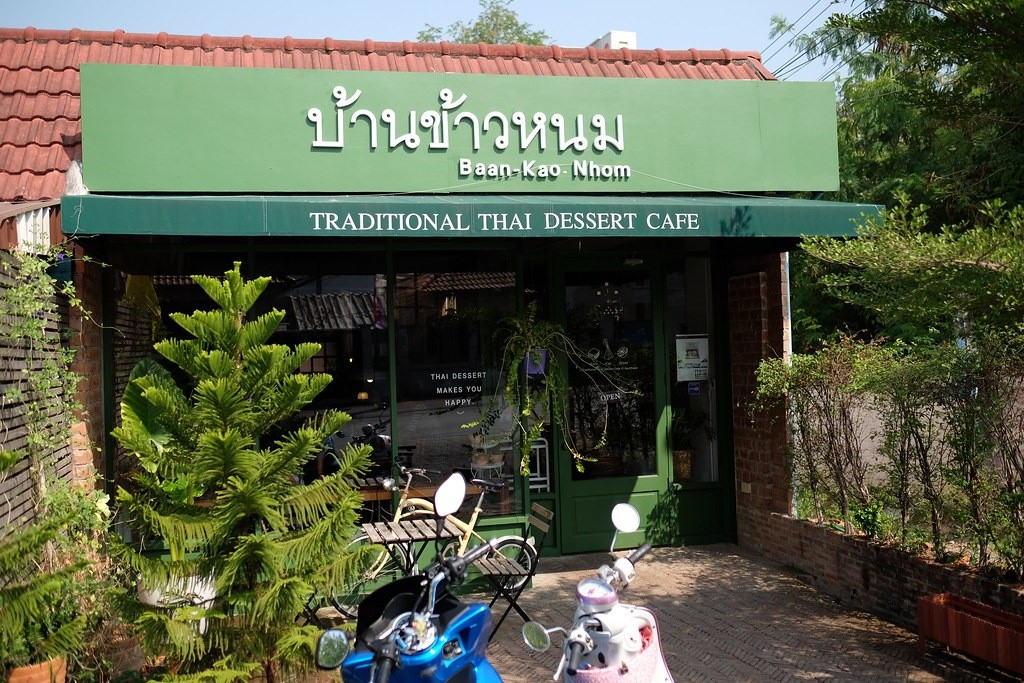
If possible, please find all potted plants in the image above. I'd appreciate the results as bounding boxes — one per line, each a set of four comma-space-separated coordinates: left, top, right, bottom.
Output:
0, 592, 78, 683
120, 360, 219, 636
461, 298, 649, 478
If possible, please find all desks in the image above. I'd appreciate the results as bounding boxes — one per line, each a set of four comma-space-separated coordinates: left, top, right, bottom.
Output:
362, 519, 465, 578
351, 476, 409, 489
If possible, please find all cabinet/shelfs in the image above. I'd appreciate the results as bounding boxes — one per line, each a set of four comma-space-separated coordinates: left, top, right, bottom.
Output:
471, 431, 552, 493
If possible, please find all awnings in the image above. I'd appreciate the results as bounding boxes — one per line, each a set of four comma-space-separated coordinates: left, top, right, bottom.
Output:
60, 193, 886, 275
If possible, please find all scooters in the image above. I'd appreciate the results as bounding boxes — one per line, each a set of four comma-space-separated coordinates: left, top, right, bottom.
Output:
521, 501, 676, 682
312, 470, 505, 683
335, 400, 391, 468
304, 430, 347, 486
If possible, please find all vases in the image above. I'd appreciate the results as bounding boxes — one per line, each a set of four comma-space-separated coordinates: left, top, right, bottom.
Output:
918, 591, 1024, 674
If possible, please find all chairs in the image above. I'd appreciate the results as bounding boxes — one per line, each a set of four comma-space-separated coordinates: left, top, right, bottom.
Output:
474, 500, 554, 643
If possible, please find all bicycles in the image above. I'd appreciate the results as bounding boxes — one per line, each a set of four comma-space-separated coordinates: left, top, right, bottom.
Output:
326, 464, 539, 619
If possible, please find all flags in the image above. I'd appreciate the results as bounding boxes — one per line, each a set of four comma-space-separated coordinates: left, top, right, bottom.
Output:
370, 297, 388, 330
